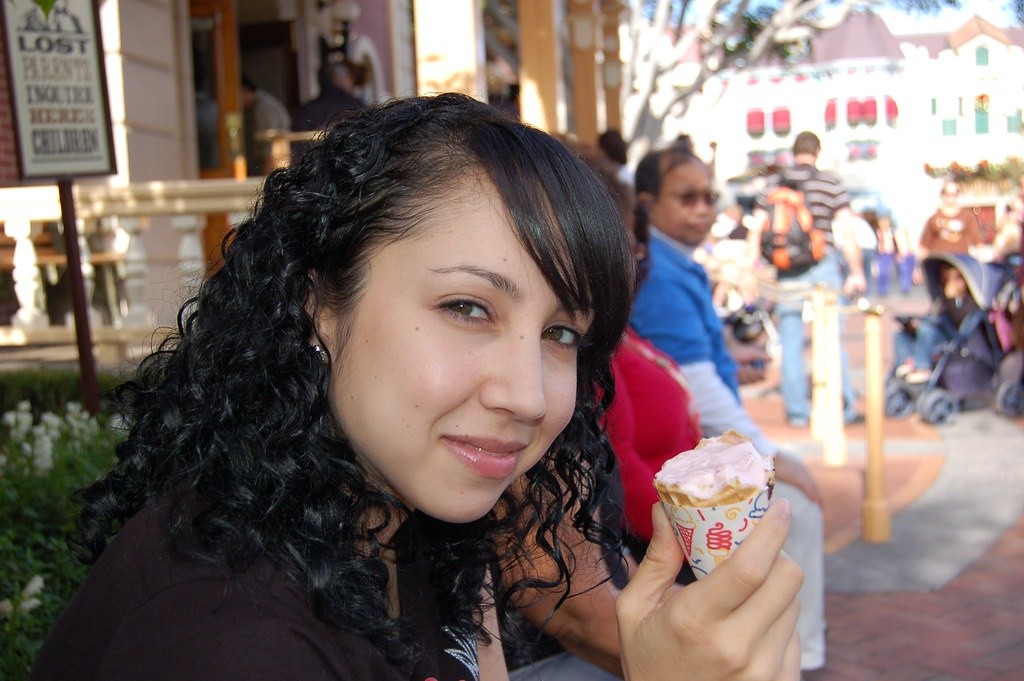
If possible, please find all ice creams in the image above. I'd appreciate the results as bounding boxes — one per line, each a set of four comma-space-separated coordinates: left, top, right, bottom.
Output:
653, 430, 775, 508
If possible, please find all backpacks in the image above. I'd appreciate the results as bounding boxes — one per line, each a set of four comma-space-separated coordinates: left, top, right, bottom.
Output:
760, 168, 826, 274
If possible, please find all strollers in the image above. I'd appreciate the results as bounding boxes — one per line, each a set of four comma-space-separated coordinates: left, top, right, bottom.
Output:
880, 251, 1024, 426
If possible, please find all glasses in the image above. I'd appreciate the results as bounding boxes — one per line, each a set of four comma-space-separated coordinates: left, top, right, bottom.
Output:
654, 189, 720, 207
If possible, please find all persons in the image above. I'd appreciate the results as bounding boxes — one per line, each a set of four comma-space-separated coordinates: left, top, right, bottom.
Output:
895, 180, 1024, 383
33, 93, 803, 681
195, 58, 371, 176
487, 128, 899, 681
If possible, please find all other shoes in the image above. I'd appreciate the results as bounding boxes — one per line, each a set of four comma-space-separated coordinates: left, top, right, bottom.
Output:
896, 362, 933, 382
848, 414, 867, 424
